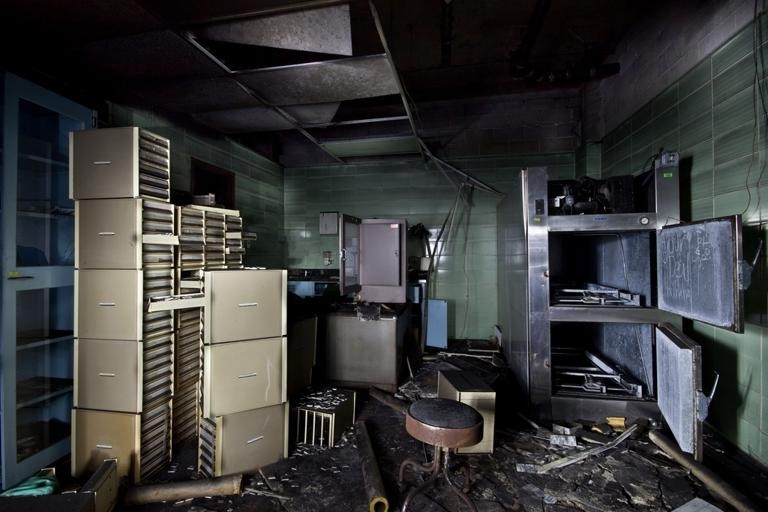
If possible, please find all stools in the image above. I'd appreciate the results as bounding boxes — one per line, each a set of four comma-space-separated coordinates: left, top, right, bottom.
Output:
396, 397, 485, 511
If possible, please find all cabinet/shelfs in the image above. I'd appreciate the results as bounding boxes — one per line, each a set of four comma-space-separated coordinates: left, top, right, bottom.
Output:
171, 204, 242, 461
68, 125, 170, 202
197, 403, 289, 480
147, 268, 287, 345
74, 269, 175, 341
74, 200, 174, 271
198, 335, 287, 420
70, 410, 170, 484
74, 335, 175, 413
